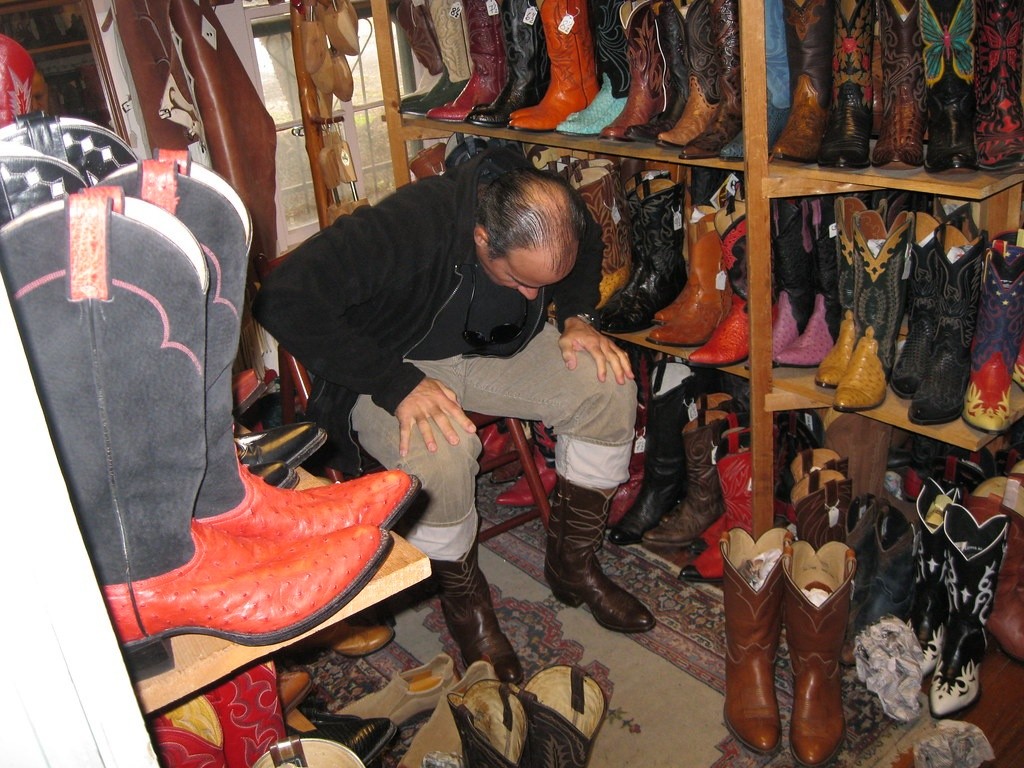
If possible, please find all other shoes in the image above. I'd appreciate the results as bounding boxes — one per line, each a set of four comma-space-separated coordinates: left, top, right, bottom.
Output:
336, 653, 456, 726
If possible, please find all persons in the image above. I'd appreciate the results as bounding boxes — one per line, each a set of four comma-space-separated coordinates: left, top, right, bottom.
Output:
250, 145, 655, 685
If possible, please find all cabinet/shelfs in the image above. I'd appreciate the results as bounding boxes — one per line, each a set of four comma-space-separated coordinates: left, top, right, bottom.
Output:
0, 0, 1024, 768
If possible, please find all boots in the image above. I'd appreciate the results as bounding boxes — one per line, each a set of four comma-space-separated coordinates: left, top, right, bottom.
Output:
430, 519, 522, 684
545, 472, 655, 632
446, 679, 528, 768
606, 350, 1024, 764
518, 664, 604, 768
396, 0, 1024, 171
479, 418, 556, 505
0, 112, 421, 681
408, 130, 1023, 434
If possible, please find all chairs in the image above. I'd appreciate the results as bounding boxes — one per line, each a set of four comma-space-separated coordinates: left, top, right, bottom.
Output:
250, 237, 554, 599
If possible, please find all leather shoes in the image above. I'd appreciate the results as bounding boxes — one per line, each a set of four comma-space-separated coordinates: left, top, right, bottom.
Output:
278, 672, 397, 763
287, 621, 395, 664
